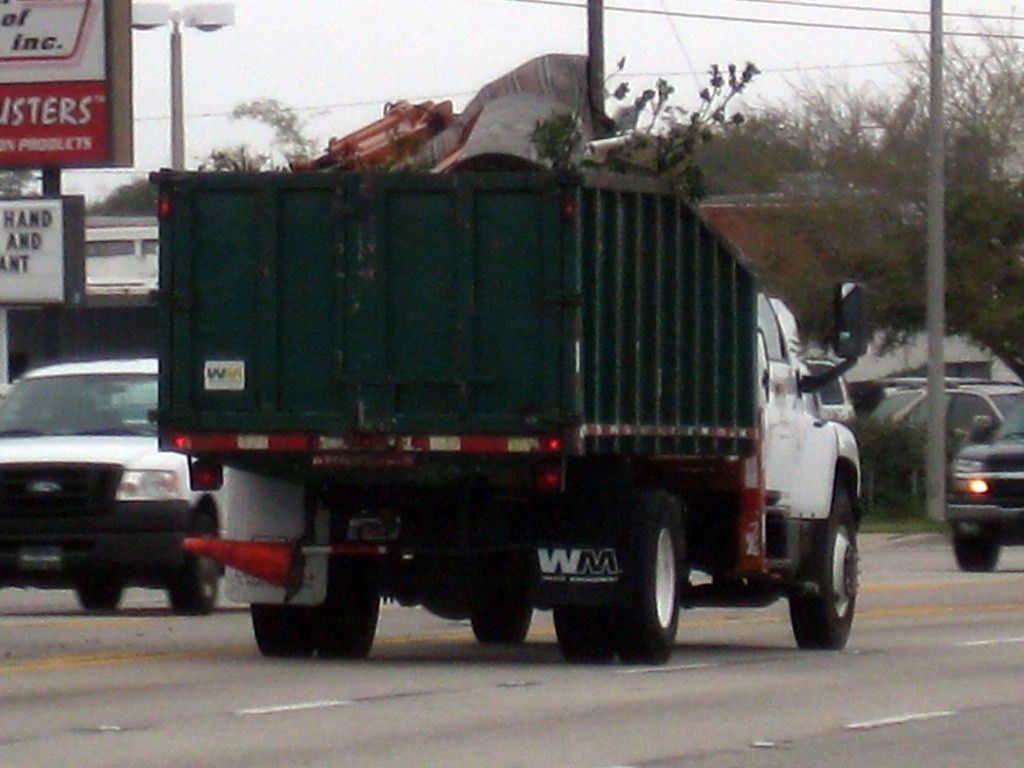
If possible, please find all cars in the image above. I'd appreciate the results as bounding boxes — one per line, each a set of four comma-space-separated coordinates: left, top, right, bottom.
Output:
0, 357, 232, 619
858, 376, 1024, 572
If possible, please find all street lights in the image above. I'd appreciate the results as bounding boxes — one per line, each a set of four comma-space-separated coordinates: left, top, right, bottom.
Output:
131, 0, 237, 171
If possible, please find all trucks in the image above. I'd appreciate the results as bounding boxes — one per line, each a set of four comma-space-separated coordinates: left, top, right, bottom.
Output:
145, 164, 871, 669
